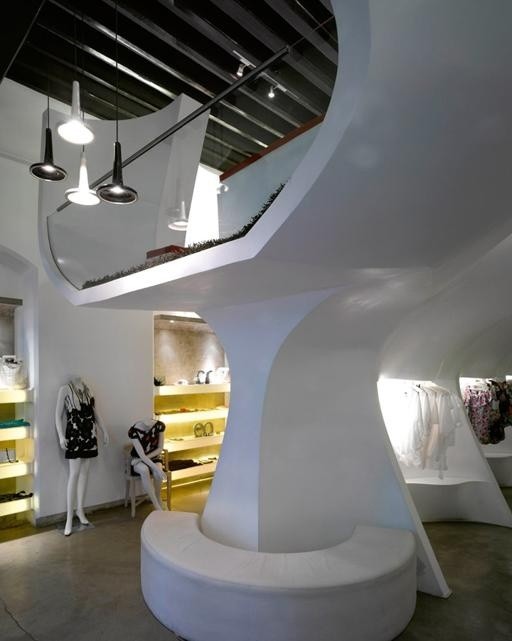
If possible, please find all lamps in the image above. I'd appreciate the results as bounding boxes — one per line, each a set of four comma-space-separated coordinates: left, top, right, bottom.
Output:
235, 50, 287, 102
28, 2, 140, 208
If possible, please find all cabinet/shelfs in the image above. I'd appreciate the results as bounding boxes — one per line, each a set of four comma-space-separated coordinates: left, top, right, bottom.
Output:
154, 378, 229, 494
1, 356, 36, 531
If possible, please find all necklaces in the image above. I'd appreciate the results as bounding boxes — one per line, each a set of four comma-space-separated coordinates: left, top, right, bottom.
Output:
70, 381, 90, 407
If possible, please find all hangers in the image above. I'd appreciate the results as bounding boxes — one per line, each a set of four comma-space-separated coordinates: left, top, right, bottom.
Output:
390, 379, 451, 400
463, 378, 511, 398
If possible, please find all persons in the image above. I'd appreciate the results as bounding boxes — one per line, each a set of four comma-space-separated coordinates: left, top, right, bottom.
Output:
54, 374, 111, 538
127, 418, 167, 512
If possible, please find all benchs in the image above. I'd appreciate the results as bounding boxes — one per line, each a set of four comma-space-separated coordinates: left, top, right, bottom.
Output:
138, 510, 419, 641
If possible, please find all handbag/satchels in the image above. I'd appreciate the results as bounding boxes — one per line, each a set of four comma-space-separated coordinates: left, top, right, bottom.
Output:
0, 447, 18, 463
0, 353, 27, 391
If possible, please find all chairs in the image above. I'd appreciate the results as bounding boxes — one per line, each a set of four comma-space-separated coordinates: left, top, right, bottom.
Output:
122, 443, 172, 518
